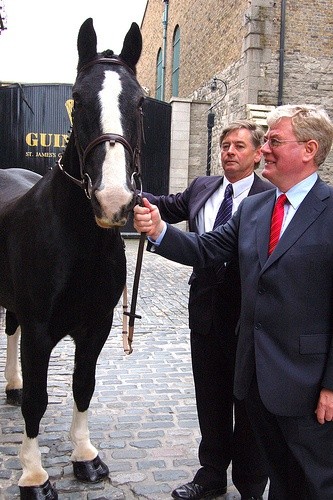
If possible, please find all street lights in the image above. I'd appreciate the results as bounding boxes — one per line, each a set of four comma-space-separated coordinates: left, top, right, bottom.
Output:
206, 77, 228, 176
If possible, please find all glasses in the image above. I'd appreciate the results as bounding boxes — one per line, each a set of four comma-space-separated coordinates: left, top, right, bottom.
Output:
262, 137, 309, 148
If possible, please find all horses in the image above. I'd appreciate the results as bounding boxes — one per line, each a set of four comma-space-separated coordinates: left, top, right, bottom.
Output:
0, 17, 147, 500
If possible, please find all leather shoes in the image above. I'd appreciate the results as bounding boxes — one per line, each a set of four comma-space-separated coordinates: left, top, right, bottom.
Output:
171, 480, 227, 500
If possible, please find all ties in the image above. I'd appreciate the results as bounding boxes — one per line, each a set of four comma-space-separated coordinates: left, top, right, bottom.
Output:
212, 184, 233, 231
267, 194, 288, 255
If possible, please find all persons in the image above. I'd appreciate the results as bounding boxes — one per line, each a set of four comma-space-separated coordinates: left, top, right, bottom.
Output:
136, 98, 332, 499
133, 121, 276, 500
